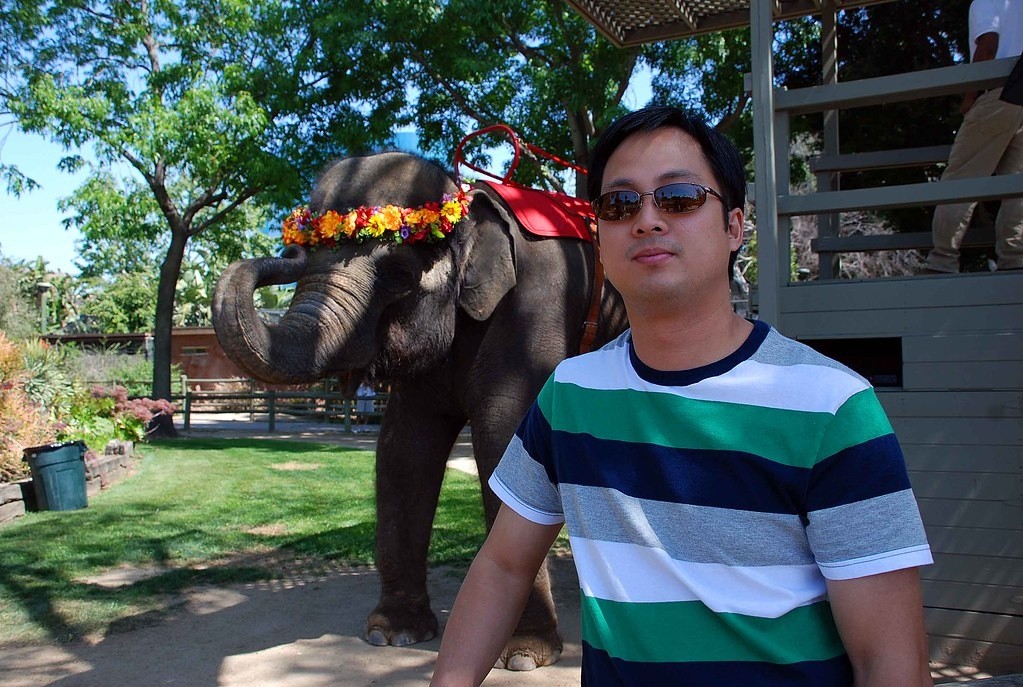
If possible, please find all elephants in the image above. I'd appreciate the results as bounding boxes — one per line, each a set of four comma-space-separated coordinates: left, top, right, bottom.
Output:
211, 152, 632, 671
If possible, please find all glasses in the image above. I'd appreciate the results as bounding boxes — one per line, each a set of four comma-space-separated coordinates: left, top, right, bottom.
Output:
591, 180, 727, 222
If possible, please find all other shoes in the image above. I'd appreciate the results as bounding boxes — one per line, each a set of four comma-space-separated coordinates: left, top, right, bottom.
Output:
924, 252, 960, 273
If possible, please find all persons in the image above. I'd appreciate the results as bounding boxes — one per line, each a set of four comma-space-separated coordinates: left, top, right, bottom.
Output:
351, 380, 376, 435
914, 2, 1020, 274
430, 103, 937, 687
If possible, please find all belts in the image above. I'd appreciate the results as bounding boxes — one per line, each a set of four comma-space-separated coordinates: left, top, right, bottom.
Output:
977, 86, 995, 98
